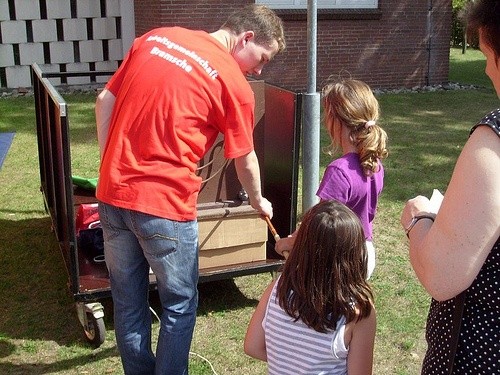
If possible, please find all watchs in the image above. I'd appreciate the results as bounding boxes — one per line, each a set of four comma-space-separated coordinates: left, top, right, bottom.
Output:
403, 212, 435, 239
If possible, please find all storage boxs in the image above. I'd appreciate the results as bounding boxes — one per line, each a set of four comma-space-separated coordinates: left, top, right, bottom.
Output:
196, 200, 269, 269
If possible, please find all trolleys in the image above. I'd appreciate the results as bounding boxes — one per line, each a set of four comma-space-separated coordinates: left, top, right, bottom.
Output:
29, 64, 304, 347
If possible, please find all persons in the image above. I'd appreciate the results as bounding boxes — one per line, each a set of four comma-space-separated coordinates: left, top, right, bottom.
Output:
243, 198, 380, 374
276, 74, 385, 281
94, 3, 286, 374
401, 0, 499, 375
